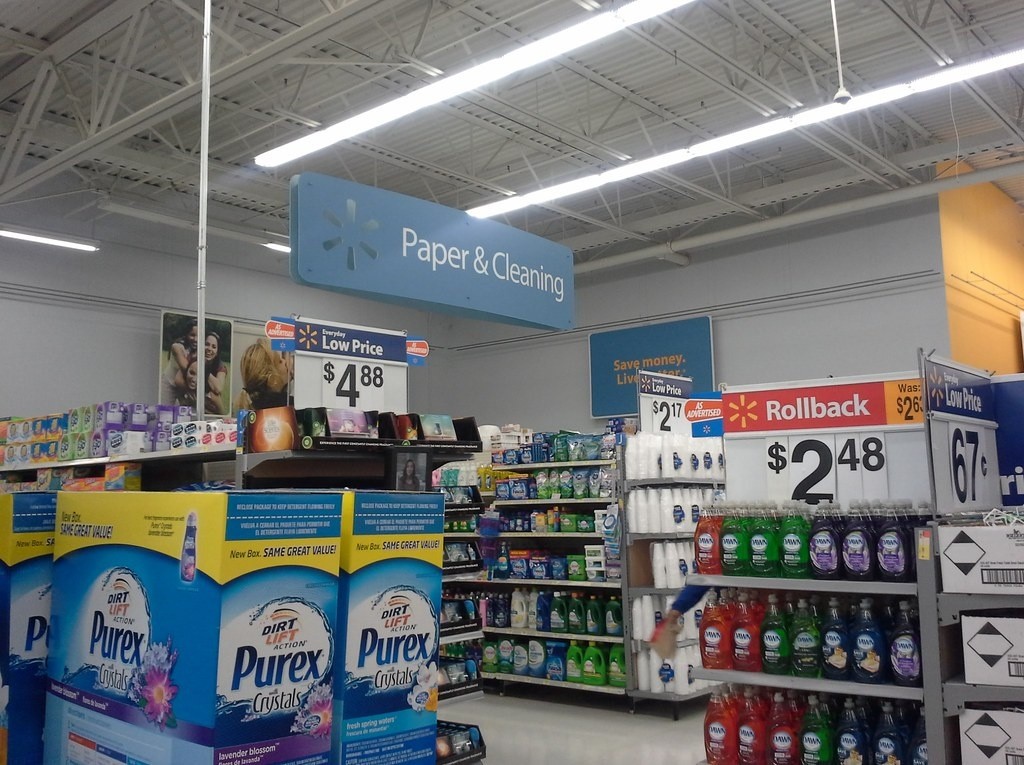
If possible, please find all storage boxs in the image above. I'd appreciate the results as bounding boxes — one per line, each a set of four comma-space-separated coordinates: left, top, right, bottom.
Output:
959, 708, 1024, 765
583, 545, 607, 582
961, 615, 1024, 687
938, 523, 1024, 595
593, 510, 608, 533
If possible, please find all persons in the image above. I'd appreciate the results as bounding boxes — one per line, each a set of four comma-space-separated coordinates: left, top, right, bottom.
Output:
343, 418, 362, 433
161, 320, 227, 414
400, 460, 420, 491
239, 340, 294, 411
666, 586, 711, 634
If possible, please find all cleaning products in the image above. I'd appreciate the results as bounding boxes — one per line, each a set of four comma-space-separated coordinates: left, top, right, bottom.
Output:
180, 512, 198, 582
624, 429, 726, 696
694, 498, 929, 765
433, 419, 627, 688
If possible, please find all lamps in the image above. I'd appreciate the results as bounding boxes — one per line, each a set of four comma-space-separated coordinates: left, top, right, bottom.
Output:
0, 222, 103, 252
466, 45, 1024, 221
253, 0, 703, 168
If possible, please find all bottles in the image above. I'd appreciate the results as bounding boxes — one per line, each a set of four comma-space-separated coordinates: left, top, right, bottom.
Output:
440, 432, 932, 765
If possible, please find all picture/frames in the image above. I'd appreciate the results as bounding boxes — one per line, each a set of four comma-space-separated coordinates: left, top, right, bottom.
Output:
158, 311, 233, 419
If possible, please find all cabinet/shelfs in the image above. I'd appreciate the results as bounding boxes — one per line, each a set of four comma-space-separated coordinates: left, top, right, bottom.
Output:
0, 315, 1024, 765
